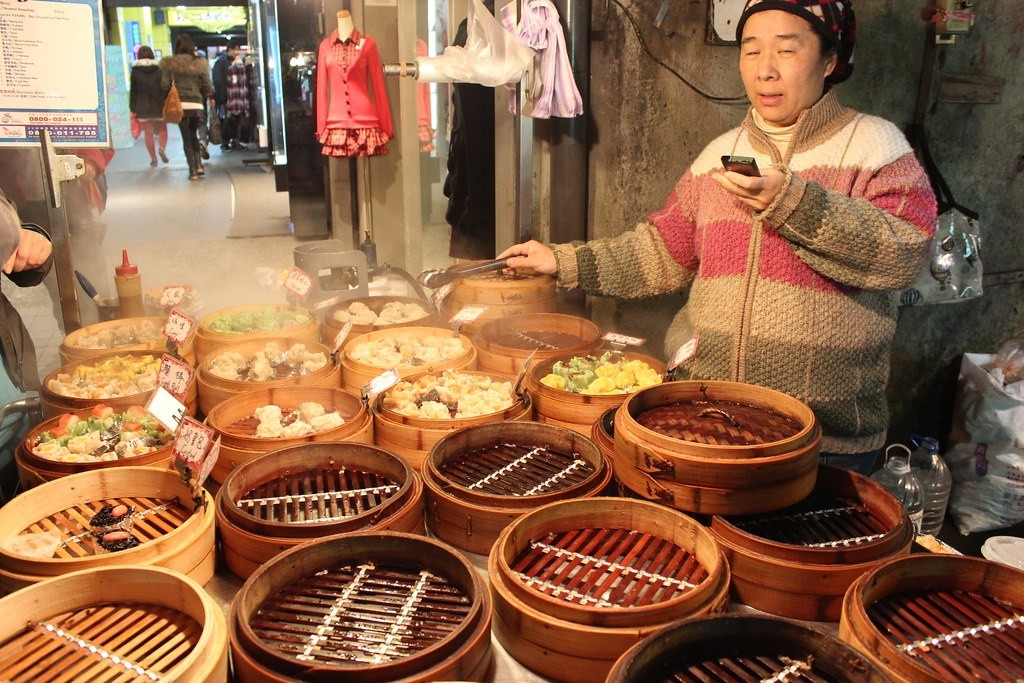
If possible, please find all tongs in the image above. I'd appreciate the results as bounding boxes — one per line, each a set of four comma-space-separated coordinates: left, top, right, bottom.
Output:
417, 254, 527, 288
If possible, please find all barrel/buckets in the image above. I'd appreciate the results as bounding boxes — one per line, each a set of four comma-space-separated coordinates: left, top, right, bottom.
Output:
97, 298, 123, 321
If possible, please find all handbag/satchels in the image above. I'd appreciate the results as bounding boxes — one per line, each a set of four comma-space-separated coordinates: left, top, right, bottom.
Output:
208, 106, 222, 145
129, 110, 140, 141
162, 75, 184, 123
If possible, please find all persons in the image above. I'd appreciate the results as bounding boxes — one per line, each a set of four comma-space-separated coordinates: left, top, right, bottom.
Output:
212, 40, 249, 152
314, 28, 395, 158
497, 0, 937, 480
161, 34, 216, 180
129, 46, 169, 167
442, 0, 497, 264
0, 179, 56, 508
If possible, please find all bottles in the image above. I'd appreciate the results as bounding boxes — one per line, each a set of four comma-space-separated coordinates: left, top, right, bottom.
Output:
870, 444, 924, 536
985, 332, 1024, 384
115, 251, 146, 317
906, 434, 952, 537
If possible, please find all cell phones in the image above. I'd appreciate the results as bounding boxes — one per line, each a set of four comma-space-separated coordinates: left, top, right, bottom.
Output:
721, 155, 761, 177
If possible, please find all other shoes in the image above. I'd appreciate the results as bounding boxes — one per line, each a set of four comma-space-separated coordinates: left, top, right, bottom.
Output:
222, 142, 249, 152
158, 150, 168, 163
197, 164, 204, 174
189, 173, 198, 180
198, 142, 210, 160
151, 160, 159, 166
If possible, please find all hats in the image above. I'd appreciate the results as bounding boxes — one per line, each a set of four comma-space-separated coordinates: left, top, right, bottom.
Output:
737, 0, 857, 85
